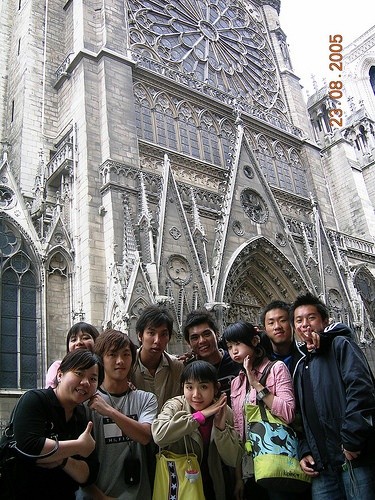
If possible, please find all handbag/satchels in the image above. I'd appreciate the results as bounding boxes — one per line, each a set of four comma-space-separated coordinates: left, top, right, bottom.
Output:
151, 431, 205, 500
244, 361, 311, 489
0, 389, 60, 476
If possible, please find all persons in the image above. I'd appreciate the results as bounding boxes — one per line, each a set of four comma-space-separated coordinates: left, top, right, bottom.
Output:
149, 359, 244, 500
127, 306, 187, 414
288, 293, 375, 500
261, 299, 322, 384
222, 320, 311, 500
87, 328, 159, 500
173, 309, 245, 410
0, 347, 105, 500
44, 321, 99, 389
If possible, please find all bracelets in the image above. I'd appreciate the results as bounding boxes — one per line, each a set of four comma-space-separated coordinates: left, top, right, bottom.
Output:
255, 388, 270, 400
58, 457, 68, 469
